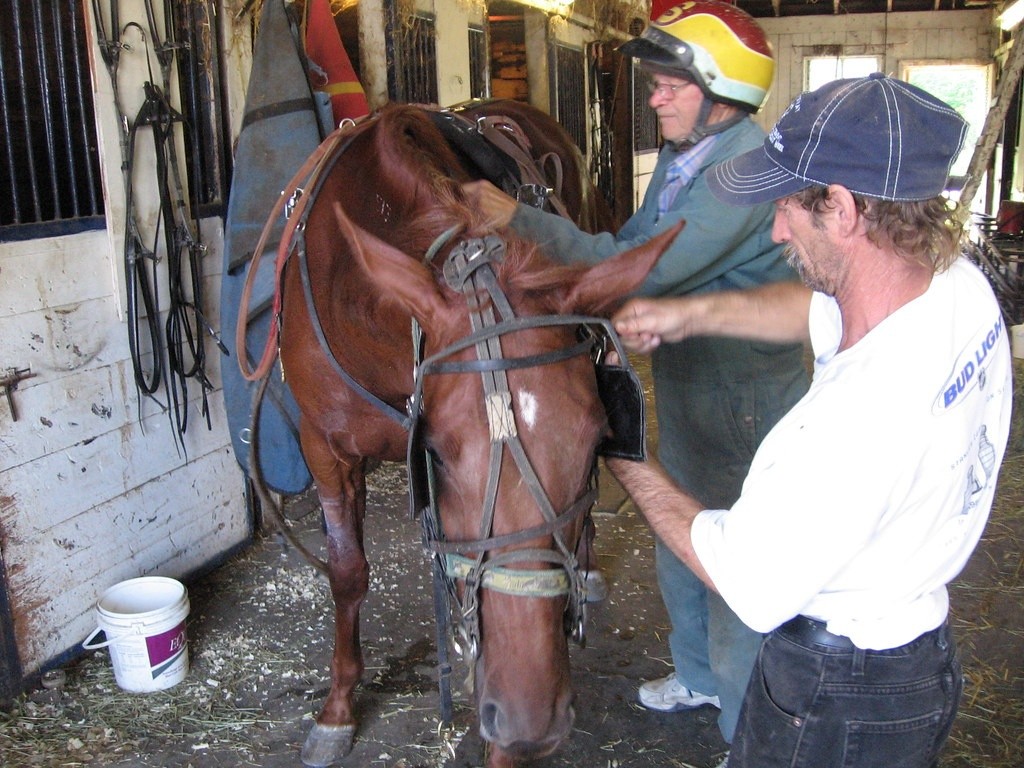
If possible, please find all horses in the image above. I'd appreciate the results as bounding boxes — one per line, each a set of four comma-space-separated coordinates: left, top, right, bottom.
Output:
277, 94, 686, 768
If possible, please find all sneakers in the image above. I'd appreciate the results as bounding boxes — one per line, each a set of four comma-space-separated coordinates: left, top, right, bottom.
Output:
638, 673, 721, 713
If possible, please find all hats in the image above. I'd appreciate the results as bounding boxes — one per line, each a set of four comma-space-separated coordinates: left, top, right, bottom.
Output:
704, 72, 970, 207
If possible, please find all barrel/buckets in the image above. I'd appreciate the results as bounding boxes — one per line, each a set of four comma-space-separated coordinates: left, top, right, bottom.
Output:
1010, 325, 1024, 359
83, 576, 193, 694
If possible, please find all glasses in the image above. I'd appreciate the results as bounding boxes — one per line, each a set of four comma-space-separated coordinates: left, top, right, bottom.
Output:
646, 80, 691, 100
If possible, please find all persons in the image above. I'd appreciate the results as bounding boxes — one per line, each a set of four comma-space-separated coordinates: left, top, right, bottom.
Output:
607, 74, 1013, 768
462, 0, 811, 768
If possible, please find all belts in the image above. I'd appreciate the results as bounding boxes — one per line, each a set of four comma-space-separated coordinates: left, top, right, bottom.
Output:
778, 613, 854, 651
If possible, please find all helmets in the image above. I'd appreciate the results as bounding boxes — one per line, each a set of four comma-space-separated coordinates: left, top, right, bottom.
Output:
613, 0, 774, 115
